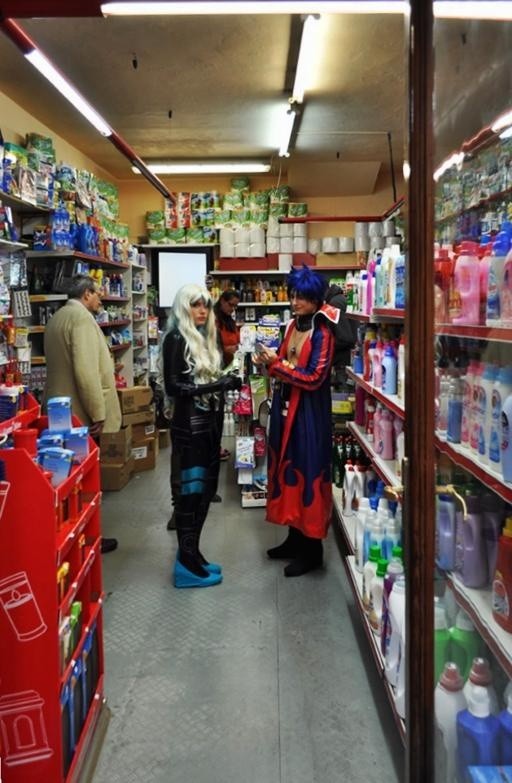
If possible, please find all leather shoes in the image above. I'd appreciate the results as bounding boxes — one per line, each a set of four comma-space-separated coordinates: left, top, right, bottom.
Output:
102, 538, 117, 553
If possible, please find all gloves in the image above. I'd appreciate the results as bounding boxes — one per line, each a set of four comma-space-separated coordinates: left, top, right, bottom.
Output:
224, 375, 242, 393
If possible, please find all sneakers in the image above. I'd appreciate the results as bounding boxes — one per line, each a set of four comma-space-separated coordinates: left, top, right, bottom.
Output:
211, 494, 221, 502
268, 544, 323, 578
220, 447, 230, 459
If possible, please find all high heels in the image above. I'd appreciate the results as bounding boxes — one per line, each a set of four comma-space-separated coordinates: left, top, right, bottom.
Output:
174, 548, 222, 588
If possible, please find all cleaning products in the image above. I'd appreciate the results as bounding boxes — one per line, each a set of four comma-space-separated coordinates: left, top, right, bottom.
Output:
335, 220, 512, 781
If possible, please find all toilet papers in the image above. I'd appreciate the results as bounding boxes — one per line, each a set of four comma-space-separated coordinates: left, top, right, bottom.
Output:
218, 218, 400, 273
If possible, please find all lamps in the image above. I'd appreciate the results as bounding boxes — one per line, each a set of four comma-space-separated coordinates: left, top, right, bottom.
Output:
0, 16, 176, 203
279, 106, 301, 157
288, 13, 320, 105
130, 158, 273, 177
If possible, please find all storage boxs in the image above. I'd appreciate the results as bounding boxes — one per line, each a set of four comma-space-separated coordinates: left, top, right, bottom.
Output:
99, 462, 133, 492
159, 428, 172, 449
132, 436, 159, 475
115, 384, 156, 424
97, 424, 134, 464
132, 424, 160, 443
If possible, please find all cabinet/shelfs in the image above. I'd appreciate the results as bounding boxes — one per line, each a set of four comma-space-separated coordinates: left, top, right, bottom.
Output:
2, 189, 30, 389
207, 260, 362, 510
1, 391, 104, 783
316, 190, 511, 783
19, 188, 159, 405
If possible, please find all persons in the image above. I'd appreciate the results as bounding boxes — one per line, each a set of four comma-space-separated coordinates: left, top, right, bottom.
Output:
43, 273, 122, 554
154, 282, 242, 588
213, 288, 244, 462
325, 284, 360, 393
250, 259, 340, 578
